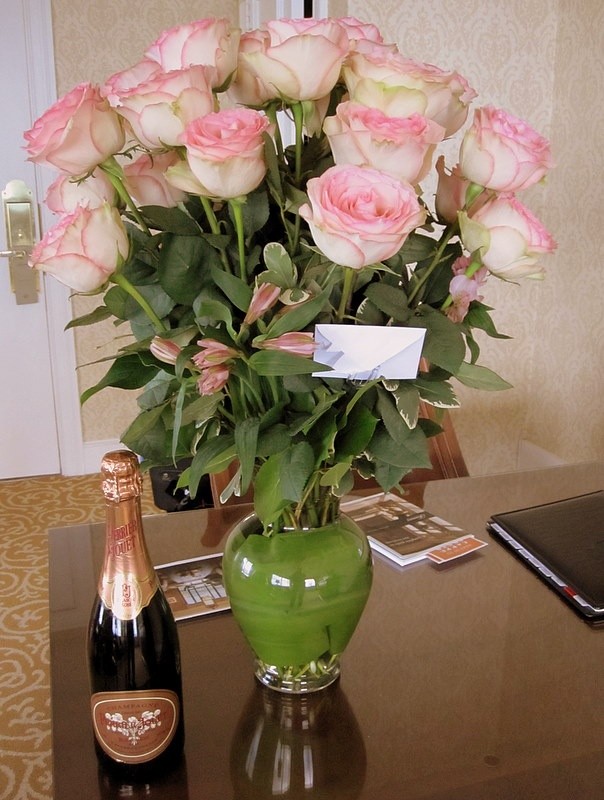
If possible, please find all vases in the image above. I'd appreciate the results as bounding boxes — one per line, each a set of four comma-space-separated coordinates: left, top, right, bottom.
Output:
222, 501, 376, 692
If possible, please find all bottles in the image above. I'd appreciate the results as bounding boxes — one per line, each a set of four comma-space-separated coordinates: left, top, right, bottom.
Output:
87, 450, 188, 785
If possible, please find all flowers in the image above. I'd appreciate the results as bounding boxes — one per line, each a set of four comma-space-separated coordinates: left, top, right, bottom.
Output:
25, 16, 557, 526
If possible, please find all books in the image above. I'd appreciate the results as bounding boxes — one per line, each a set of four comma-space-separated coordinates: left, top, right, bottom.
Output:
485, 489, 604, 630
338, 490, 473, 566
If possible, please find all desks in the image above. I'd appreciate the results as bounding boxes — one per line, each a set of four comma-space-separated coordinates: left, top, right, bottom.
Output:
47, 464, 604, 800
